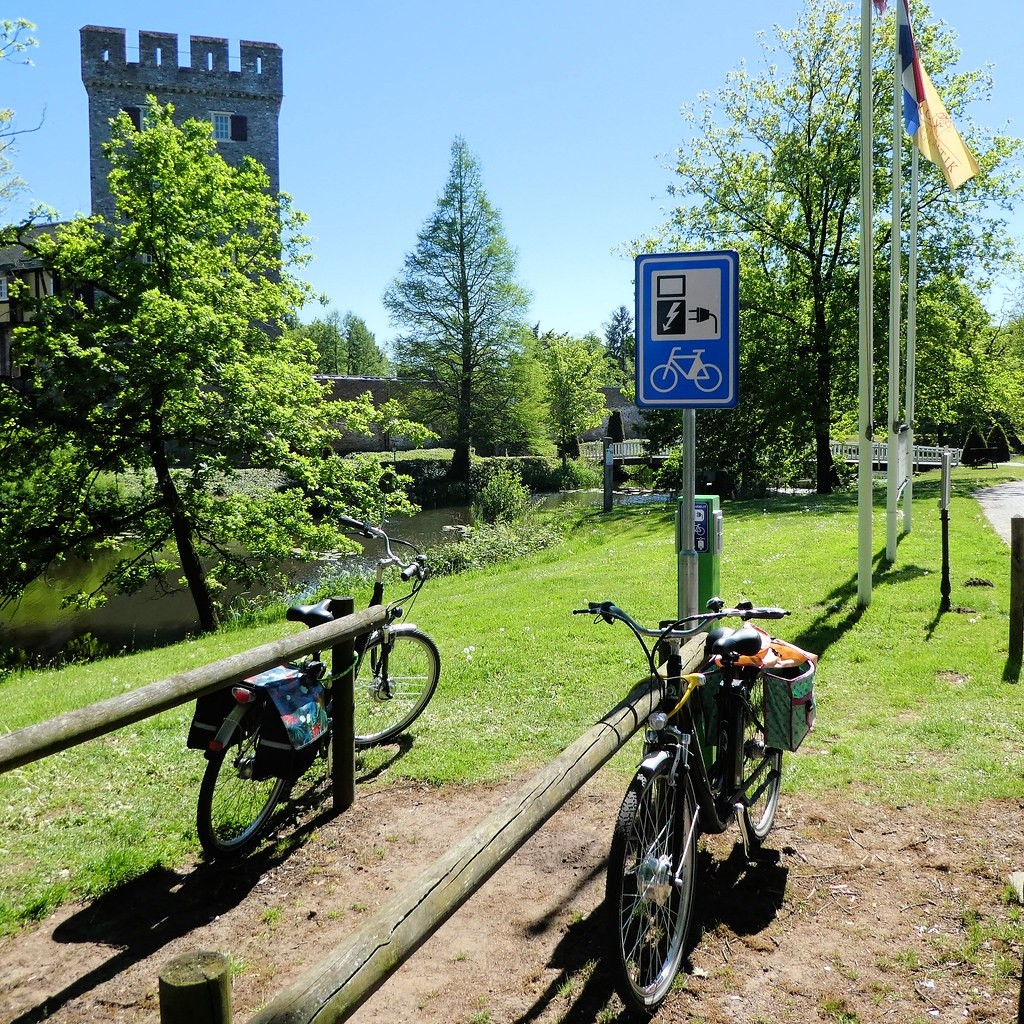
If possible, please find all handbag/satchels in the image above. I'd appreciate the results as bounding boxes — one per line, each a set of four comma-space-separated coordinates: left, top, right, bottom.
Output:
692, 639, 826, 748
188, 667, 331, 780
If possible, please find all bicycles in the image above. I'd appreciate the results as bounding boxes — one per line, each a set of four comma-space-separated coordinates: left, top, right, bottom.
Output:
570, 596, 785, 1013
193, 514, 443, 857
